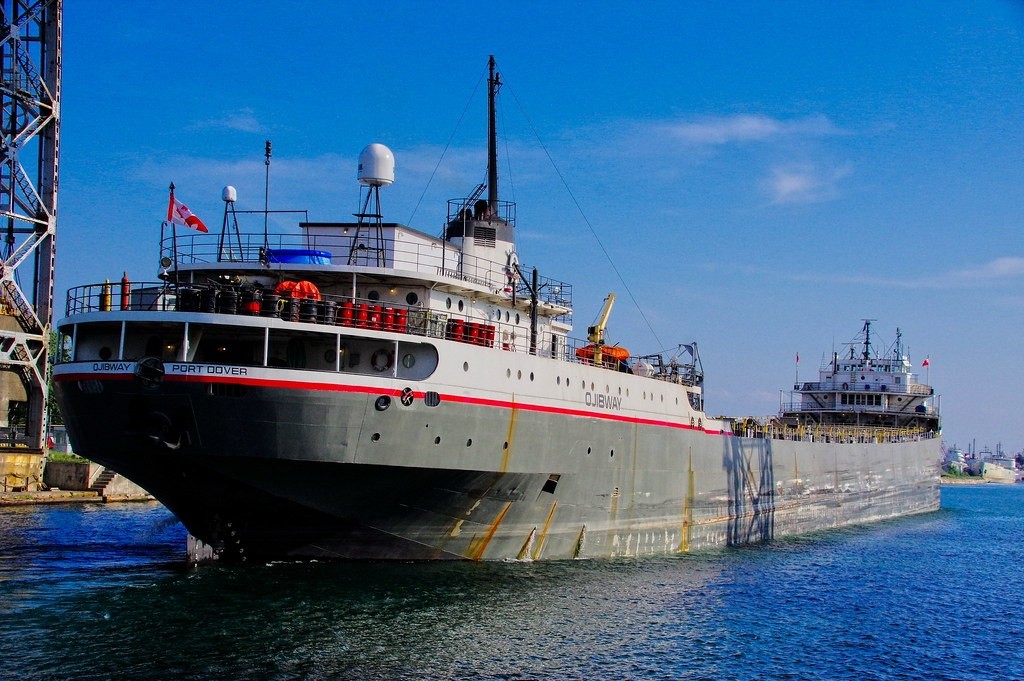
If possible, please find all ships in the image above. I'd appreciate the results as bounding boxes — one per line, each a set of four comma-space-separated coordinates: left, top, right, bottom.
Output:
49, 52, 942, 561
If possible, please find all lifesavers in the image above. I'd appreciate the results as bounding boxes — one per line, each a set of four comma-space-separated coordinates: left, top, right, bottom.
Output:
370, 348, 395, 373
509, 253, 520, 273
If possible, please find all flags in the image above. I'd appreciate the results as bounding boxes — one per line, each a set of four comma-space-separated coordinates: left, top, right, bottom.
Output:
168, 193, 210, 235
922, 359, 929, 368
796, 355, 799, 362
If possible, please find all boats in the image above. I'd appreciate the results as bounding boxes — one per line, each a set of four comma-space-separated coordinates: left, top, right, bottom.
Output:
941, 438, 1024, 484
